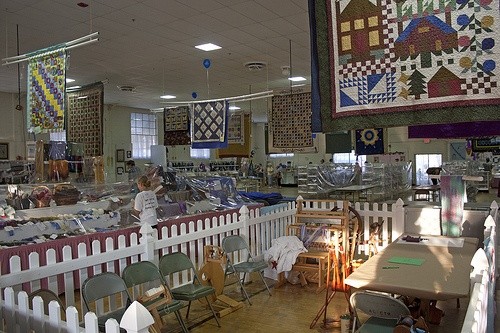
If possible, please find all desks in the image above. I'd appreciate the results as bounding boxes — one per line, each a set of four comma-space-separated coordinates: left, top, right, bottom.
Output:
274, 251, 329, 290
336, 184, 376, 202
413, 186, 440, 201
343, 233, 480, 312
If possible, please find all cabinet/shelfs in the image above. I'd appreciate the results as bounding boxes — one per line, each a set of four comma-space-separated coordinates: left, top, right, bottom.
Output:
297, 166, 318, 195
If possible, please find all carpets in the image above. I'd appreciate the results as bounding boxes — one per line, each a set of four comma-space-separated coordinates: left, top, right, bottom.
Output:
265, 90, 318, 154
26, 43, 104, 162
306, 1, 500, 153
160, 99, 252, 158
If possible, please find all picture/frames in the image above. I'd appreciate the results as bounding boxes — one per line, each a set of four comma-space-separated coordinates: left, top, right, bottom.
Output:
0, 142, 10, 160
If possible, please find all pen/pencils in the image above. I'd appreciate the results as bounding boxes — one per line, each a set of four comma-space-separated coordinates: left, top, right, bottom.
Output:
382, 266, 400, 269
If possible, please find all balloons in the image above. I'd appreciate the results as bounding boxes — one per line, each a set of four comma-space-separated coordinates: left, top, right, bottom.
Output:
203, 59, 210, 69
192, 92, 198, 99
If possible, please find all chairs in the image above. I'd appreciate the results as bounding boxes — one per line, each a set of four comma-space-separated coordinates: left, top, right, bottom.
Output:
222, 235, 273, 306
27, 289, 66, 318
122, 260, 188, 333
349, 290, 414, 333
81, 271, 155, 333
159, 252, 222, 331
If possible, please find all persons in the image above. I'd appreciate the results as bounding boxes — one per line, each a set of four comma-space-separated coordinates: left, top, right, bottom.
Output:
267, 163, 275, 188
483, 157, 493, 189
277, 163, 283, 189
131, 175, 159, 229
354, 162, 361, 183
254, 163, 264, 187
199, 161, 206, 172
209, 162, 217, 171
328, 159, 335, 166
319, 158, 327, 165
